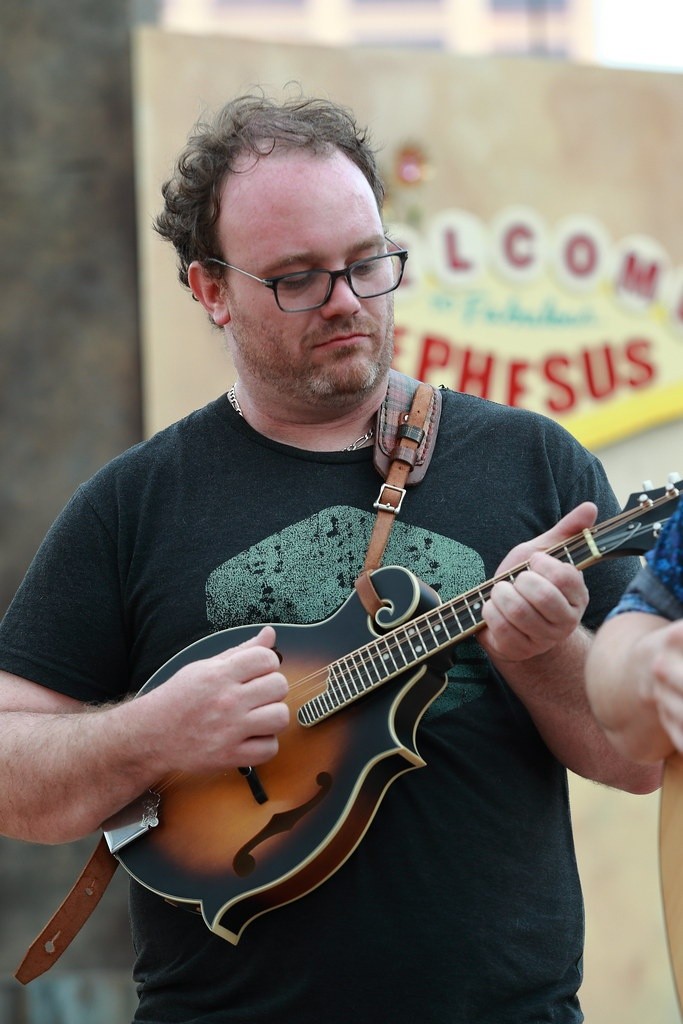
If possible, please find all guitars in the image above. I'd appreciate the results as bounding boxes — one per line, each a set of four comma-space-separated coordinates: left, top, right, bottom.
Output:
93, 474, 683, 950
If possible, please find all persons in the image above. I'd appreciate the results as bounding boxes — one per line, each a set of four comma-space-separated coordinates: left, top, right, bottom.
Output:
0, 93, 662, 1024
584, 478, 683, 1024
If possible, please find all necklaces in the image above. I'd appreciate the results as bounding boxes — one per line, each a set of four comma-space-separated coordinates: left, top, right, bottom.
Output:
230, 383, 376, 450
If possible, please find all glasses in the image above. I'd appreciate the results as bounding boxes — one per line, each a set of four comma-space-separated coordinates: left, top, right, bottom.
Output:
207, 236, 408, 312
